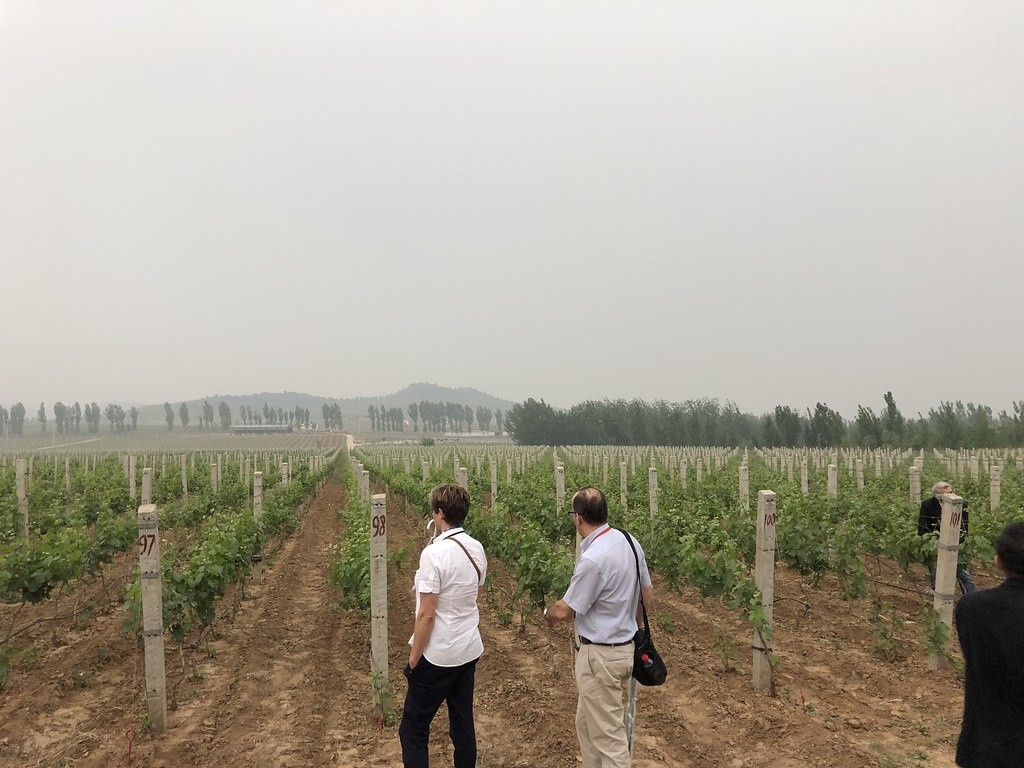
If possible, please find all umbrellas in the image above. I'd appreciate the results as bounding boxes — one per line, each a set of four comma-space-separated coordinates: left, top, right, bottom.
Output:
426, 519, 438, 546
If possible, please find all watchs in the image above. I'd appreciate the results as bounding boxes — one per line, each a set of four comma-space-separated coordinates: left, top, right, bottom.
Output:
543, 607, 550, 621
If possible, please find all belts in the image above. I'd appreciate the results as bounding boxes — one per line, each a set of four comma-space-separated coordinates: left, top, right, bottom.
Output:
578, 636, 631, 646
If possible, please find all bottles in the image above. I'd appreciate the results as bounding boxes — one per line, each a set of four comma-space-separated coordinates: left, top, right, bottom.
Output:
641, 654, 665, 685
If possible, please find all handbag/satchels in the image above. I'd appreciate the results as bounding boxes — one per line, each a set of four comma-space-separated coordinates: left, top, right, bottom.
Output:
632, 629, 668, 686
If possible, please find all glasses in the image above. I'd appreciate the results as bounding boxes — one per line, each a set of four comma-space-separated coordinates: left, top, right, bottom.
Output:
568, 511, 582, 518
428, 508, 435, 514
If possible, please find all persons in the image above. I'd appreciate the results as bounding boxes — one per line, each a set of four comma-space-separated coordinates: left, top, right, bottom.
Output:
544, 487, 653, 768
398, 482, 488, 768
954, 522, 1024, 768
918, 482, 978, 595
